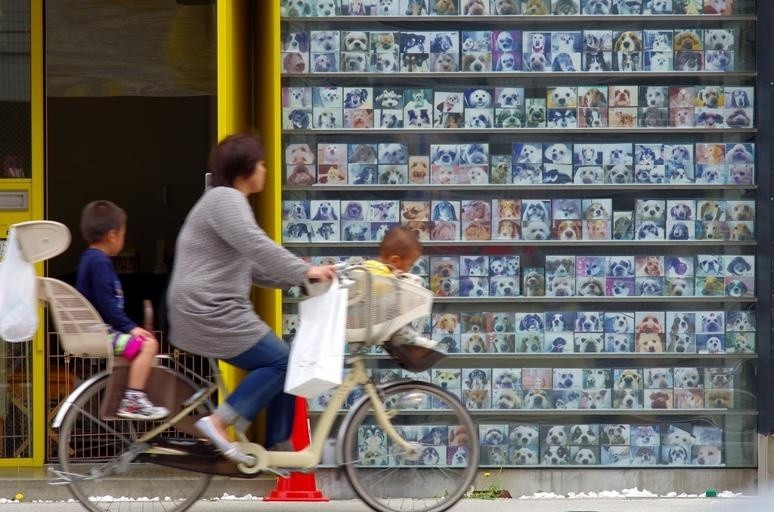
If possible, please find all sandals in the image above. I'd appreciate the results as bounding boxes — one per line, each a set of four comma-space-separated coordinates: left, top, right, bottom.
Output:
193, 416, 238, 459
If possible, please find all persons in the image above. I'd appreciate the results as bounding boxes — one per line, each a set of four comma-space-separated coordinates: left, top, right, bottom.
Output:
166, 133, 338, 462
75, 200, 170, 419
349, 226, 439, 347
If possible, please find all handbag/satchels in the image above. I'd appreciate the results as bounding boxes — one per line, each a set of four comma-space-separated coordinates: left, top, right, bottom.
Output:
0, 228, 38, 343
283, 272, 348, 399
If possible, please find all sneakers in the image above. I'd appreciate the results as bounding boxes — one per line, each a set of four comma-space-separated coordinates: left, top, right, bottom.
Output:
114, 390, 170, 419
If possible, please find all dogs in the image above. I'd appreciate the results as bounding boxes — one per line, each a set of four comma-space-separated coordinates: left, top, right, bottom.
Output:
281, 0, 756, 466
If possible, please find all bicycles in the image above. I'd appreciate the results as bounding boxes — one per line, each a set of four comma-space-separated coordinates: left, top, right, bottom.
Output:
52, 264, 481, 512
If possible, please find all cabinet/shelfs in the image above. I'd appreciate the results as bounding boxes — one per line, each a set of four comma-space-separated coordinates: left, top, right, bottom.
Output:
280, 0, 758, 469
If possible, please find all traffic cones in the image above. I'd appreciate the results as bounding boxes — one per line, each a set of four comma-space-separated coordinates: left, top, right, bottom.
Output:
262, 396, 330, 501
